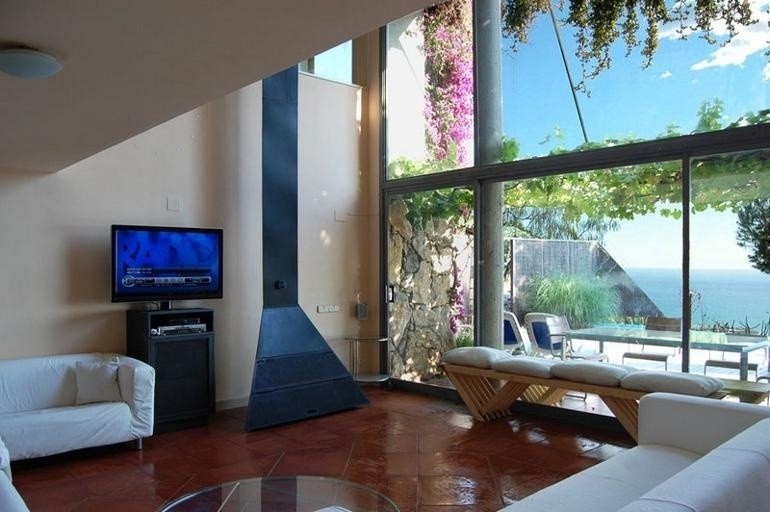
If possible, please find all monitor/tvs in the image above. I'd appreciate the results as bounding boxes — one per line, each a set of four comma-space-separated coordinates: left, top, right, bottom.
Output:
109, 223, 223, 310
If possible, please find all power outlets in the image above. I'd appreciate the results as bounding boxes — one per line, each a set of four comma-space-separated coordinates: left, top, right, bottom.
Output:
334, 208, 351, 222
167, 195, 183, 211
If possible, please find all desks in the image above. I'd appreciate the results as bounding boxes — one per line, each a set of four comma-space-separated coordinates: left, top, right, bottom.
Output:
156, 475, 400, 512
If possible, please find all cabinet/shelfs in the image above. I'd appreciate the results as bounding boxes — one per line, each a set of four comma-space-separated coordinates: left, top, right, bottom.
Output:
344, 334, 391, 386
126, 306, 217, 434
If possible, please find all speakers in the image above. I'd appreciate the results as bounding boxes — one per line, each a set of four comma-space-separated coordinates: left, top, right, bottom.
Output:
356, 303, 368, 320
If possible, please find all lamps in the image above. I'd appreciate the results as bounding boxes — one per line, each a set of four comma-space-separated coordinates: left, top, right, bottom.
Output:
1, 46, 63, 80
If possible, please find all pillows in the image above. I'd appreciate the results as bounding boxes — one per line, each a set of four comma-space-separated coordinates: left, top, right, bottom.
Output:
439, 342, 724, 447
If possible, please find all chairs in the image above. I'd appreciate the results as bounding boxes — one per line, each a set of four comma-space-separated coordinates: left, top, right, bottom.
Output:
503, 310, 770, 384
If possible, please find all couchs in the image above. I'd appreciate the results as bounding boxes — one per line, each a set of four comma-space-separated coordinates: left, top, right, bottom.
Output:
0, 350, 156, 462
494, 391, 770, 512
